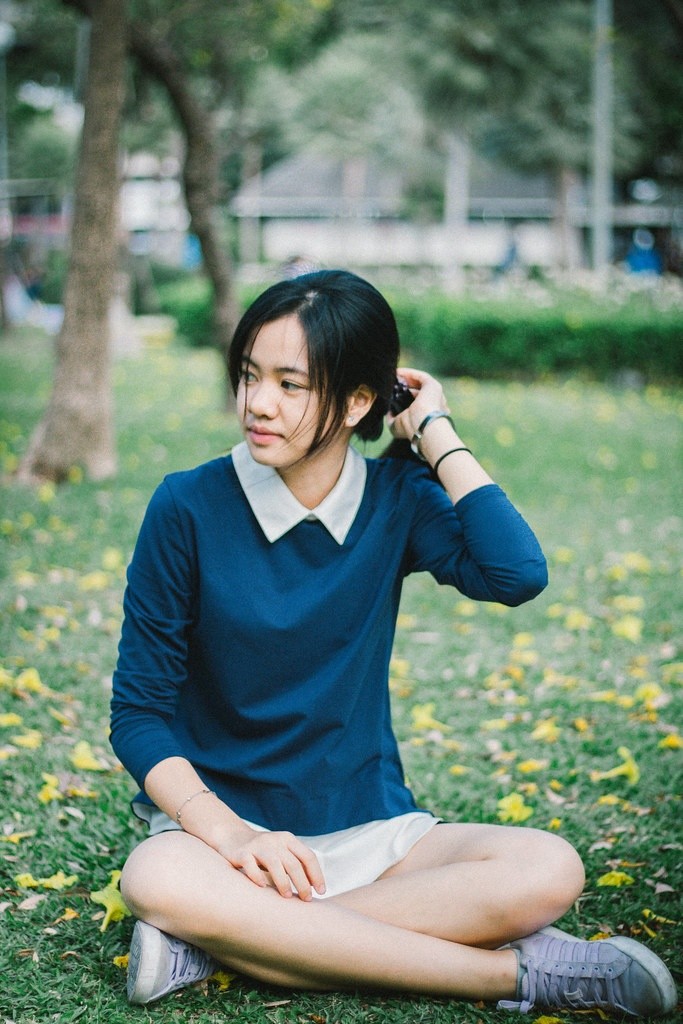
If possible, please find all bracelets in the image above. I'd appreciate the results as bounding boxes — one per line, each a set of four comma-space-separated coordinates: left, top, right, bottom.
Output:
409, 410, 456, 462
433, 446, 472, 484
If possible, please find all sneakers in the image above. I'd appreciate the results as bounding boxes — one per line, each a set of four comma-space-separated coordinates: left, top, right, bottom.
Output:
126, 918, 225, 1005
496, 924, 678, 1020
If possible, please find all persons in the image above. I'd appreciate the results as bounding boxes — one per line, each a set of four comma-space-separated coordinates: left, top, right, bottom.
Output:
110, 268, 680, 1022
